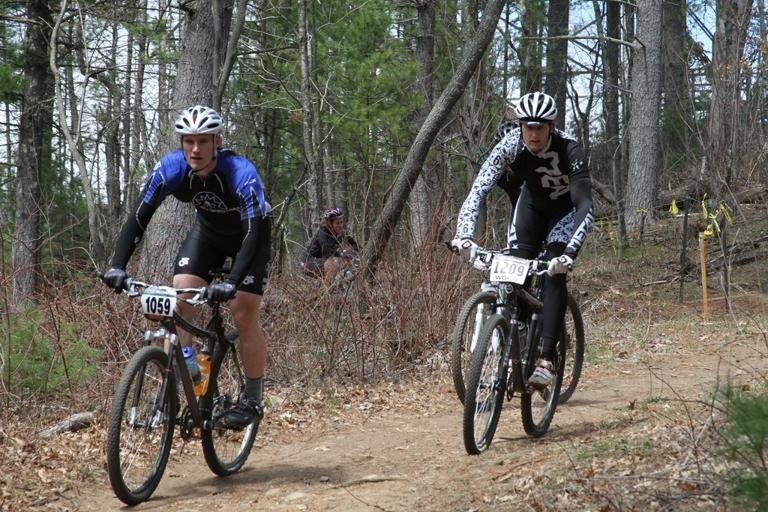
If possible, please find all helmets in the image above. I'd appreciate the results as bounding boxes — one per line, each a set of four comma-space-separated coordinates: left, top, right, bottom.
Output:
175, 104, 222, 136
515, 91, 558, 125
323, 207, 344, 220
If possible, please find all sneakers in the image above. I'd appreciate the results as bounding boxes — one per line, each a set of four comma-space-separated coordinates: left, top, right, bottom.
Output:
528, 358, 556, 384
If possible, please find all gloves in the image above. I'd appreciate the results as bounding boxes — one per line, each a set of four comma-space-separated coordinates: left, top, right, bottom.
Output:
205, 282, 236, 302
451, 238, 478, 264
546, 255, 573, 277
103, 267, 126, 294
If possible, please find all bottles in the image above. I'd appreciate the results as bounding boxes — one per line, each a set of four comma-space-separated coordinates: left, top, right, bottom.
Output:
182, 346, 202, 387
194, 347, 212, 396
518, 321, 527, 355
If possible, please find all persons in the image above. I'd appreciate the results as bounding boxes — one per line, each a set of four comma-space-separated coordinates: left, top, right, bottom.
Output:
449, 88, 598, 392
98, 103, 275, 430
297, 204, 361, 296
490, 117, 565, 361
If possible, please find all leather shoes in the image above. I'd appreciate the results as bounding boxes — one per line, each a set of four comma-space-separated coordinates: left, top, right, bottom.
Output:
227, 401, 265, 424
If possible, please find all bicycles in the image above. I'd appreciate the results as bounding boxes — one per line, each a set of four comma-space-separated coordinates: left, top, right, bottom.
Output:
95, 270, 264, 505
444, 238, 585, 454
